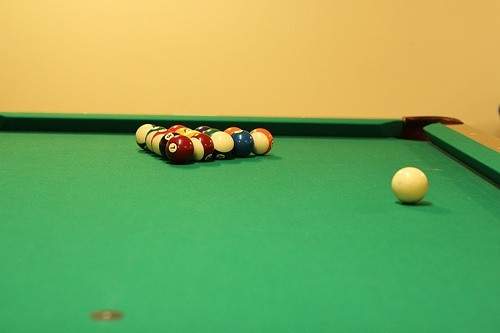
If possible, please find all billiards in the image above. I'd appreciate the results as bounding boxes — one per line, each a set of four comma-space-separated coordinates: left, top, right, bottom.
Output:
135, 123, 275, 166
391, 167, 429, 205
